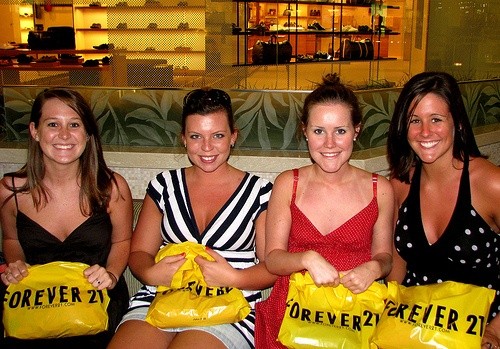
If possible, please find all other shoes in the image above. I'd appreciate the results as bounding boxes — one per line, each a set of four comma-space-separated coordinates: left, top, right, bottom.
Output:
297, 51, 327, 61
232, 22, 242, 32
358, 23, 373, 32
253, 8, 320, 30
16, 0, 191, 66
375, 24, 393, 32
306, 22, 325, 29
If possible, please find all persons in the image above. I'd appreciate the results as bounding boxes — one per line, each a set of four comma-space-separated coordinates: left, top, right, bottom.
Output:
0, 86, 133, 349
379, 70, 500, 349
106, 85, 274, 349
255, 72, 396, 349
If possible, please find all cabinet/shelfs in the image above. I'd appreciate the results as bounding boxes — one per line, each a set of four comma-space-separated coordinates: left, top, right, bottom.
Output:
0, 0, 400, 86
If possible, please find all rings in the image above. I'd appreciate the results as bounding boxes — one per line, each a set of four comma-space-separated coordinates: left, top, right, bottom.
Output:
95, 278, 101, 285
4, 272, 12, 278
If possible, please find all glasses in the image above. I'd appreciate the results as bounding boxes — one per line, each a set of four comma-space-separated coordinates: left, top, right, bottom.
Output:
183, 88, 231, 109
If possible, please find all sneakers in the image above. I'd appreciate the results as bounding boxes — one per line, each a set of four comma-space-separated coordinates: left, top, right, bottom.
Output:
341, 24, 358, 31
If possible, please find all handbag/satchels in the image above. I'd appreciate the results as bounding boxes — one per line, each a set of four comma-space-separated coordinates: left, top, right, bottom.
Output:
276, 267, 496, 348
3, 261, 110, 338
340, 35, 374, 59
144, 240, 251, 329
